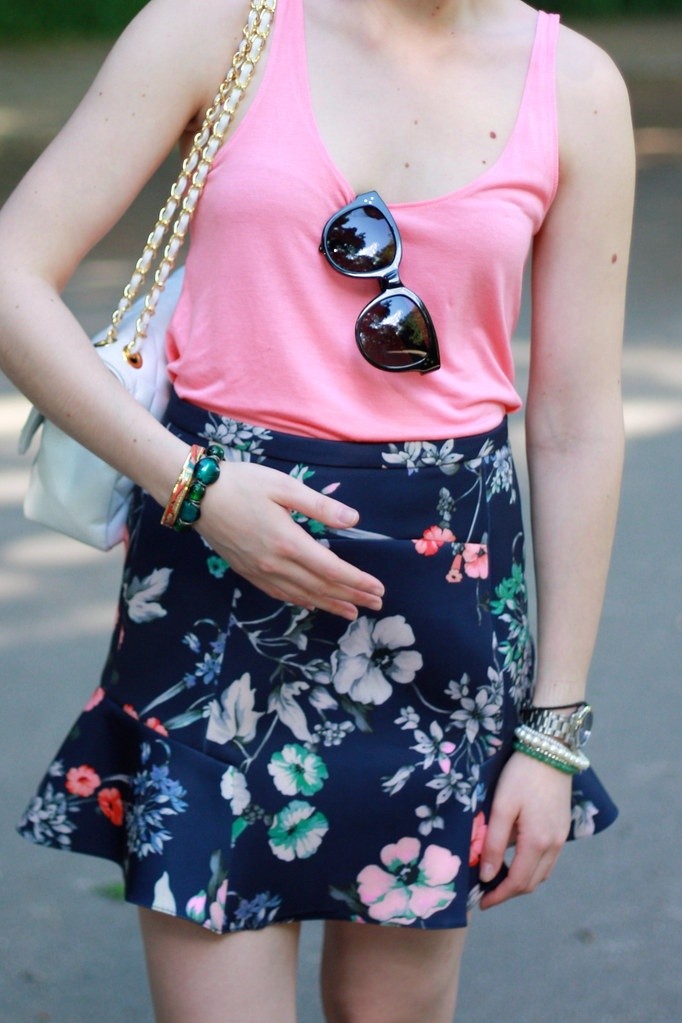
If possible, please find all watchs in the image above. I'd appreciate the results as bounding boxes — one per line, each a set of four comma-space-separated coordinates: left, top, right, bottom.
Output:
524, 705, 593, 751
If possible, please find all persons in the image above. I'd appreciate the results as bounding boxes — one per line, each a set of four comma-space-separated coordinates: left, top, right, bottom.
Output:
0, 0, 636, 1023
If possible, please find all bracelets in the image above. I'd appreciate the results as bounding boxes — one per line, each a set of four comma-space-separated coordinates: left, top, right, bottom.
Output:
512, 702, 590, 775
161, 445, 225, 532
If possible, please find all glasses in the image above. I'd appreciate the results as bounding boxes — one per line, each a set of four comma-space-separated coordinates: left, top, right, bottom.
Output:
318, 190, 440, 376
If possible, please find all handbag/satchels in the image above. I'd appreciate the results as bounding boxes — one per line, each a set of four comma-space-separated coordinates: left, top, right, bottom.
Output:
17, 265, 184, 552
